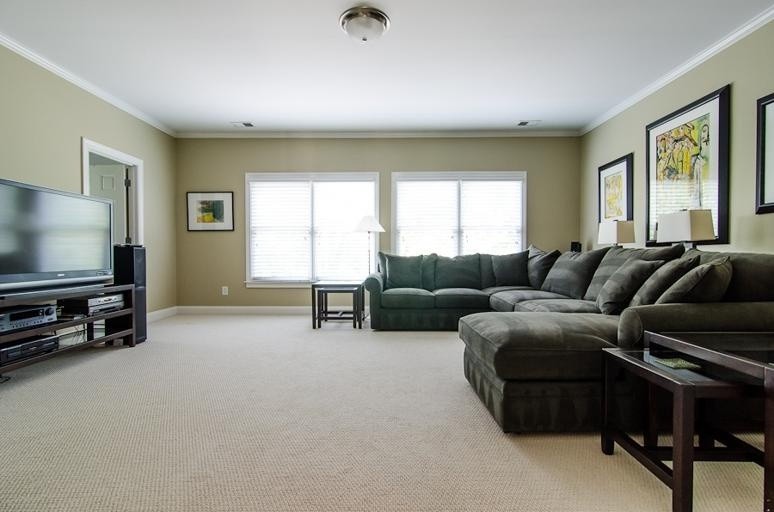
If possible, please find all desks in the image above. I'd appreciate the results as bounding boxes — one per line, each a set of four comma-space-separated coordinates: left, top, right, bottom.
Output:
311, 280, 369, 330
598, 327, 772, 512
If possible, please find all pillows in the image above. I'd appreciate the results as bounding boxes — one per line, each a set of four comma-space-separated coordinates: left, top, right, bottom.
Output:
488, 249, 531, 288
596, 253, 666, 315
655, 253, 733, 304
382, 252, 424, 290
628, 255, 703, 307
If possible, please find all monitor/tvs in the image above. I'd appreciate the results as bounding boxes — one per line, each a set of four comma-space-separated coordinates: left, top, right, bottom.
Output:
0, 177, 115, 292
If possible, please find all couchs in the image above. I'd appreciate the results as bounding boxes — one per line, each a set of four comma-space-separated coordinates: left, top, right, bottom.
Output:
366, 242, 773, 438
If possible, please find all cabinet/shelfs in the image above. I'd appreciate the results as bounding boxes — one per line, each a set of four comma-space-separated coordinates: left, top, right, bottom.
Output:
0, 283, 136, 383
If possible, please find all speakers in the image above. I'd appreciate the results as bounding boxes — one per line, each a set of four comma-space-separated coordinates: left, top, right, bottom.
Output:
104, 242, 148, 345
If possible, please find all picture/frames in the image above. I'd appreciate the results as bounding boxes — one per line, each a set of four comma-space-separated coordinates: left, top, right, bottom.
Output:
185, 190, 235, 232
755, 90, 774, 217
596, 151, 635, 221
643, 81, 730, 244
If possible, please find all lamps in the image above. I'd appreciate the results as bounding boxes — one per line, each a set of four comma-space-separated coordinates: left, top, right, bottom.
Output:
654, 209, 716, 249
596, 218, 636, 248
337, 4, 390, 44
354, 216, 386, 274
